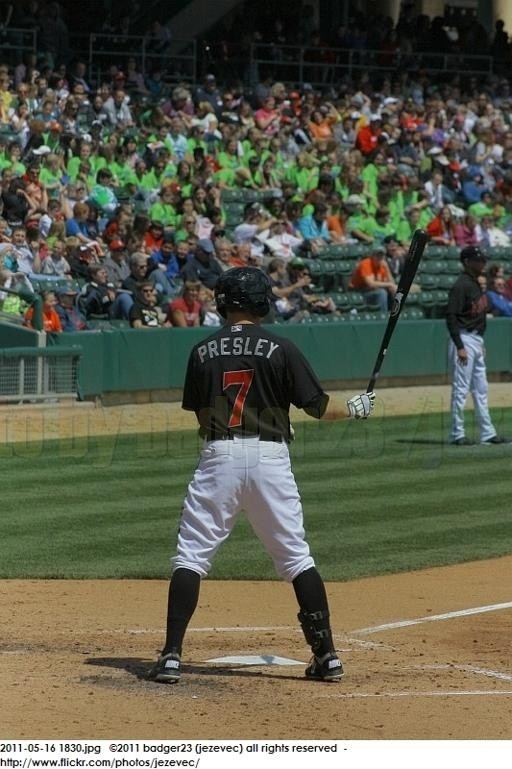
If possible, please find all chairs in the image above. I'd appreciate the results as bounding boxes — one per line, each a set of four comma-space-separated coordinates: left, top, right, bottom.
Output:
15, 184, 510, 329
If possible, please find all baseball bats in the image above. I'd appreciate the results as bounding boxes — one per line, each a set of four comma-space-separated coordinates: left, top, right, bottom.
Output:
367, 228, 427, 394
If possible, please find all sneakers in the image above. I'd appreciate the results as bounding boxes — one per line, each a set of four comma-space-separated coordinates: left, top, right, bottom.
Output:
305, 654, 345, 682
454, 437, 477, 446
144, 653, 182, 686
482, 436, 512, 444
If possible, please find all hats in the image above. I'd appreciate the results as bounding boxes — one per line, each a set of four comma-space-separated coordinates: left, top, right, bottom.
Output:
459, 246, 485, 258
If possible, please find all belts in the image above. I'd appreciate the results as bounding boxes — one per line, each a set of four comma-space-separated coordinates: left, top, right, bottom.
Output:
205, 433, 282, 443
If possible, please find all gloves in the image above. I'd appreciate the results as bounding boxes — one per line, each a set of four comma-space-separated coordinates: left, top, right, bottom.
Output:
349, 392, 375, 420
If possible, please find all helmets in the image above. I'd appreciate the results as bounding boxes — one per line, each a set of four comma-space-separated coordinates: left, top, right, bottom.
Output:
213, 266, 281, 318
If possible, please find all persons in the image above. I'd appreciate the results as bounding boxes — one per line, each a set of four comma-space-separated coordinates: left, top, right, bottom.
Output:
146, 265, 375, 682
0, 0, 512, 336
445, 246, 503, 443
